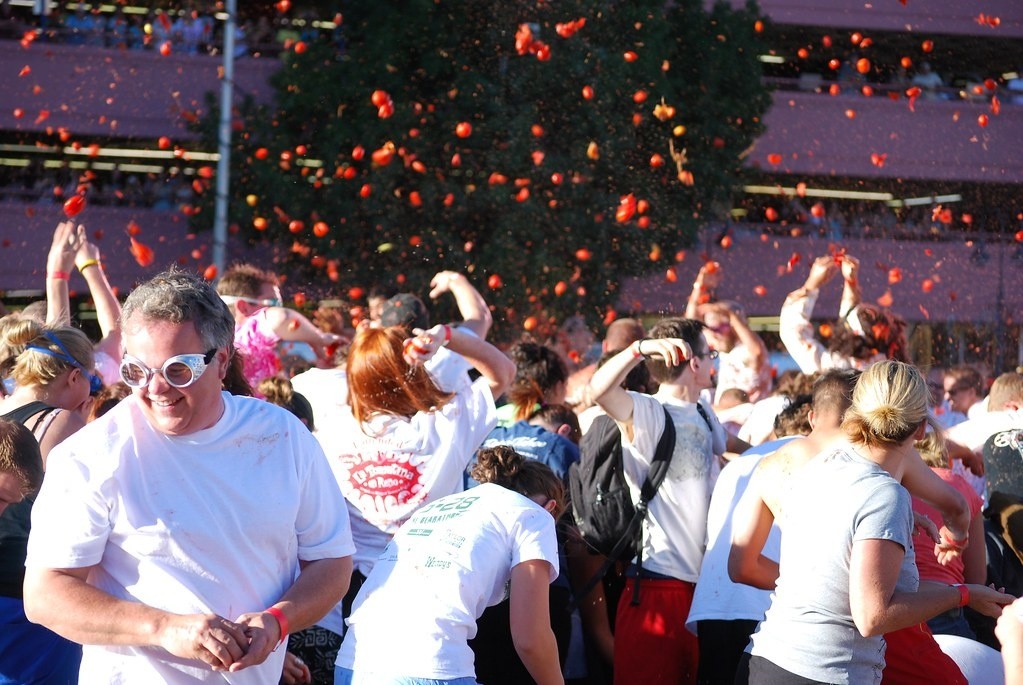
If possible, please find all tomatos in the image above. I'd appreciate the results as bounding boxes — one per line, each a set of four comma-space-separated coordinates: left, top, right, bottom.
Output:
0, 0, 1023, 364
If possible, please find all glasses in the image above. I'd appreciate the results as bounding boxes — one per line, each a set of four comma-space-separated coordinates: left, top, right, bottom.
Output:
263, 298, 284, 308
946, 384, 976, 395
90, 375, 102, 397
695, 350, 719, 360
119, 353, 211, 389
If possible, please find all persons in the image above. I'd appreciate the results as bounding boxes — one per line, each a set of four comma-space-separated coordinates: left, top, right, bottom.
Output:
0, 220, 1023, 685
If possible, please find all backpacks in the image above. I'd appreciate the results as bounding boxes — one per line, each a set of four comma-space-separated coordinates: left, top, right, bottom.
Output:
569, 399, 712, 562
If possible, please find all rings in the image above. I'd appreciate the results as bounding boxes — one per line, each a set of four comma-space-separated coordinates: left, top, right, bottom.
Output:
922, 515, 927, 519
295, 656, 304, 666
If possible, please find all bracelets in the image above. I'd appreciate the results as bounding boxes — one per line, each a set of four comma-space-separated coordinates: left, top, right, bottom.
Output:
950, 582, 970, 608
310, 330, 325, 346
630, 342, 646, 361
45, 272, 70, 282
441, 325, 452, 347
639, 337, 652, 359
844, 278, 859, 287
448, 273, 460, 290
944, 530, 968, 546
78, 259, 101, 272
267, 608, 290, 652
693, 282, 708, 291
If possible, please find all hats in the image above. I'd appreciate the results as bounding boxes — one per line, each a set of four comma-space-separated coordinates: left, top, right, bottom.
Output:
381, 293, 430, 335
